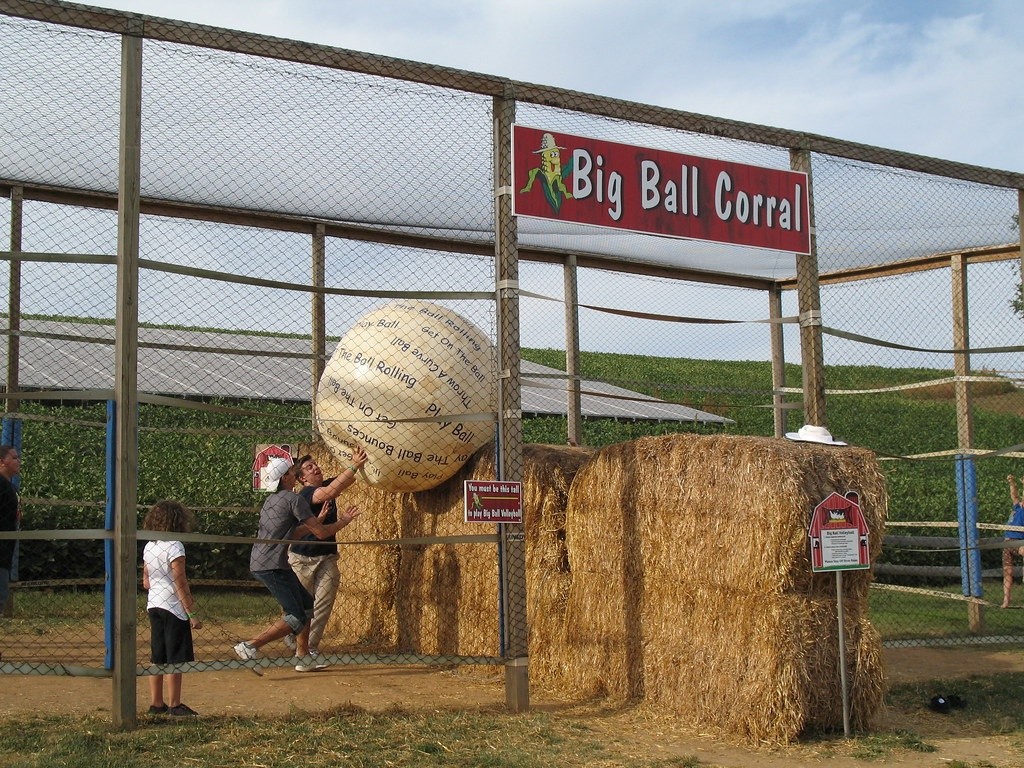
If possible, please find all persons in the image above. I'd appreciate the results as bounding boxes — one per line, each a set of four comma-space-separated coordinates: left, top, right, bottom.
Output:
142, 498, 204, 717
1000, 473, 1024, 609
233, 445, 371, 676
0, 443, 22, 616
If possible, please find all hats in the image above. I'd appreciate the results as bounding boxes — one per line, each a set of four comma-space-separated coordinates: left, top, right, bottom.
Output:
265, 458, 292, 492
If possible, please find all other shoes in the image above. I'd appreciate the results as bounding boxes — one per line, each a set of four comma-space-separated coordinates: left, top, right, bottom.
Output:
310, 651, 319, 659
284, 635, 297, 653
148, 703, 168, 714
167, 703, 198, 716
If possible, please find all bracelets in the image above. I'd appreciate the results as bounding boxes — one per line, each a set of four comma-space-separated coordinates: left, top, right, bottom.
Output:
348, 463, 358, 474
187, 610, 200, 618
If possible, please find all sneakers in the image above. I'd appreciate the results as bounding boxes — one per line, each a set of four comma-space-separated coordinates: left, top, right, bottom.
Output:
234, 642, 264, 676
295, 655, 320, 671
